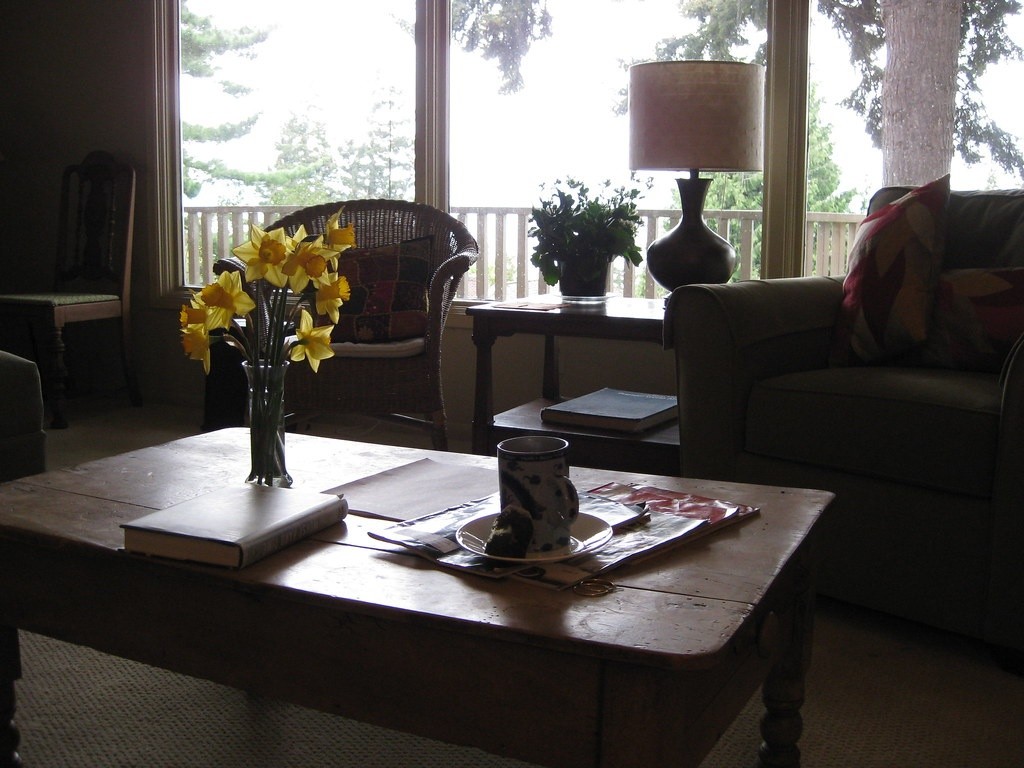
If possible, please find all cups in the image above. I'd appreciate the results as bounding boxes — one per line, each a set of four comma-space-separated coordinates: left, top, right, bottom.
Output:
497, 436, 579, 554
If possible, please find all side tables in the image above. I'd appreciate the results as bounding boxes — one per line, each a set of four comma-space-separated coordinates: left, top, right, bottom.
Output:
466, 293, 681, 476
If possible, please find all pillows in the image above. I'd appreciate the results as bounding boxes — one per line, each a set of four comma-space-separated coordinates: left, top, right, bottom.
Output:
918, 266, 1023, 373
831, 179, 949, 369
301, 235, 438, 343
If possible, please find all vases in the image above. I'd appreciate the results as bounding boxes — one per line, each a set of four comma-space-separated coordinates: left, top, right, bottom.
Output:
242, 359, 293, 487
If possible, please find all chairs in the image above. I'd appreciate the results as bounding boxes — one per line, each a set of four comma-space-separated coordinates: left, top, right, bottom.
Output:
0, 150, 143, 429
205, 199, 479, 451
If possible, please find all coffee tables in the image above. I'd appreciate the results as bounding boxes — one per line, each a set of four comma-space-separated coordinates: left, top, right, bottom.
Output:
1, 427, 836, 768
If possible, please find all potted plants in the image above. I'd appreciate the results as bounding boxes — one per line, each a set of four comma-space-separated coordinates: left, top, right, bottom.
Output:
529, 172, 653, 306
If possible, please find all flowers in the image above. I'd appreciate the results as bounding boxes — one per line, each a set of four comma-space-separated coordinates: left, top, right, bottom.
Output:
179, 205, 357, 484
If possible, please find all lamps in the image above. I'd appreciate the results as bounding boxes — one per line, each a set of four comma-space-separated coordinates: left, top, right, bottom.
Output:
630, 61, 767, 292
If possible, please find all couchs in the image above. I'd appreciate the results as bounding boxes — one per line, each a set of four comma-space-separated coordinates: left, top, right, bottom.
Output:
660, 187, 1024, 676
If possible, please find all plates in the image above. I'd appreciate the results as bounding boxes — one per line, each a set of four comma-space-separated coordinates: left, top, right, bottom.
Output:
456, 511, 614, 565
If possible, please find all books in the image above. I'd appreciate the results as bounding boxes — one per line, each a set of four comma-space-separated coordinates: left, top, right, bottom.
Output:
119, 481, 350, 569
540, 387, 678, 433
